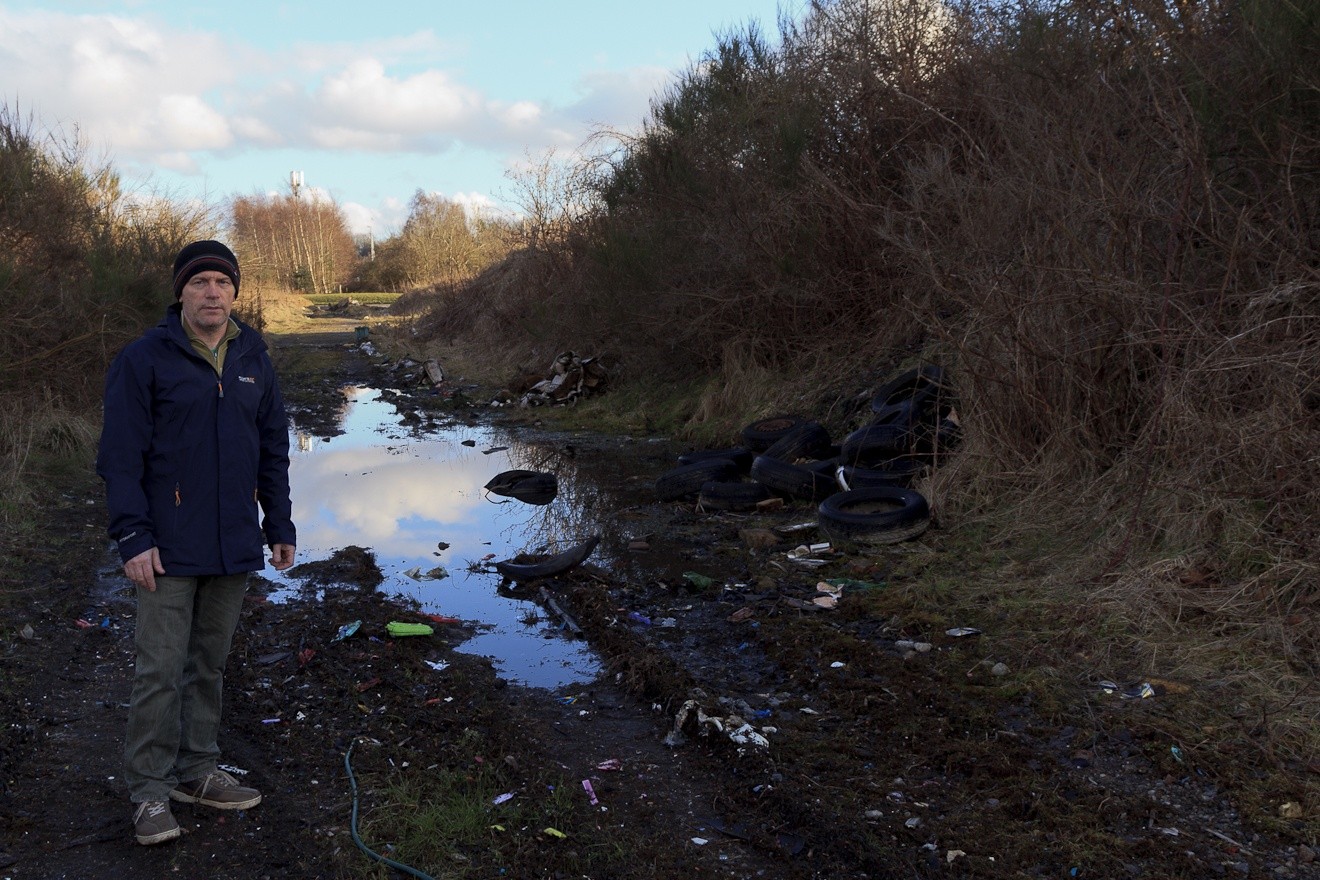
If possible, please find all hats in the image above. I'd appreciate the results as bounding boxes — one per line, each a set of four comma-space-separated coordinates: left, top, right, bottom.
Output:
174, 240, 240, 299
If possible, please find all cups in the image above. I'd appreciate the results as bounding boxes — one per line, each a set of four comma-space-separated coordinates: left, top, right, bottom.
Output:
787, 544, 809, 559
810, 541, 831, 554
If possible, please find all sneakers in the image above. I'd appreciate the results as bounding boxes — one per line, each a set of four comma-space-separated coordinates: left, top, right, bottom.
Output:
169, 769, 262, 810
133, 800, 180, 845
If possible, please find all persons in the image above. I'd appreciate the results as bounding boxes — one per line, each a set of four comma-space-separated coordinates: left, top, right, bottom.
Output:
96, 242, 299, 843
